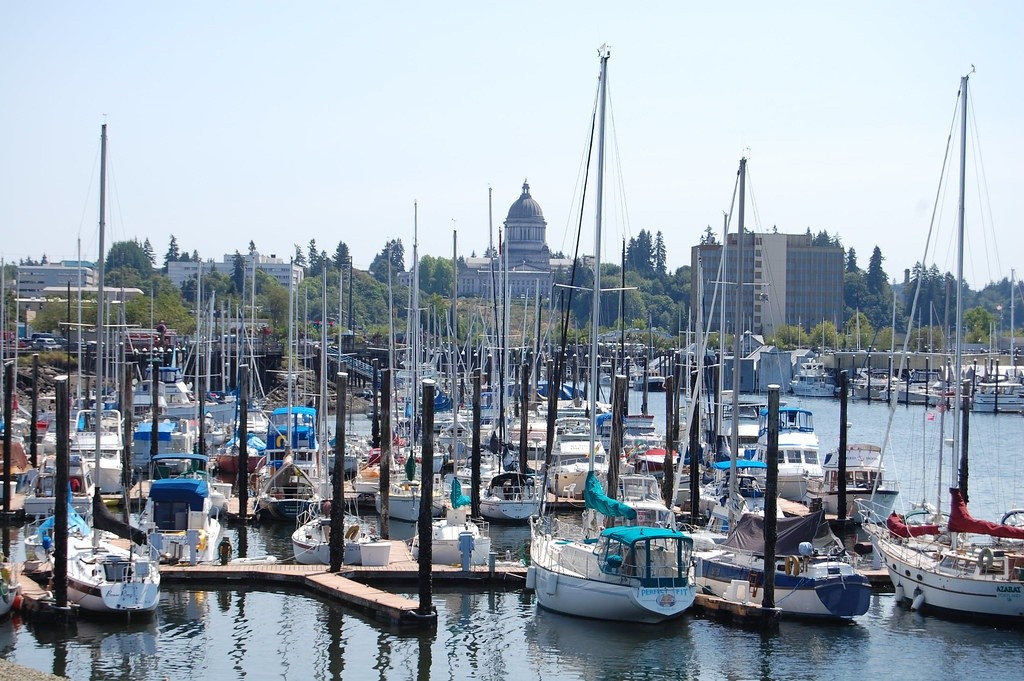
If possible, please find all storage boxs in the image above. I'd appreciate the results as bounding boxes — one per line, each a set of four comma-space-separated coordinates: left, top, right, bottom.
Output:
359, 542, 392, 566
0, 481, 18, 500
212, 482, 234, 500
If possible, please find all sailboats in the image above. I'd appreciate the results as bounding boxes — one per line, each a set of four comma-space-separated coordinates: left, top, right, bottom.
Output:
0, 46, 1024, 629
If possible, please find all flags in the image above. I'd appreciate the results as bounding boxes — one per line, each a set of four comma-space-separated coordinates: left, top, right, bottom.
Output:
936, 403, 943, 413
928, 411, 935, 420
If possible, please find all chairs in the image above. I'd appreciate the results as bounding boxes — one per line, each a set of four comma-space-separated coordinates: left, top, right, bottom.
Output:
562, 484, 576, 499
440, 483, 453, 500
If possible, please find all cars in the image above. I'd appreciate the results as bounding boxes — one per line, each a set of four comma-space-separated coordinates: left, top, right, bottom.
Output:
32, 338, 62, 350
64, 342, 87, 351
